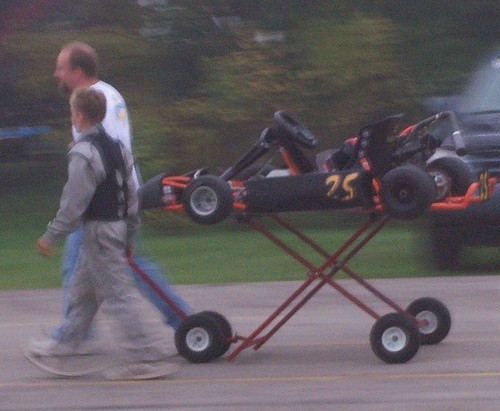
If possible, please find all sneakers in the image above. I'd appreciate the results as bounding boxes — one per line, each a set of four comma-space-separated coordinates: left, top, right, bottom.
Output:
105, 361, 173, 381
29, 338, 75, 356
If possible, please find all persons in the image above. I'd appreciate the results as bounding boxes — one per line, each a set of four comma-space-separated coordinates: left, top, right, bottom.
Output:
45, 41, 193, 340
28, 88, 176, 379
